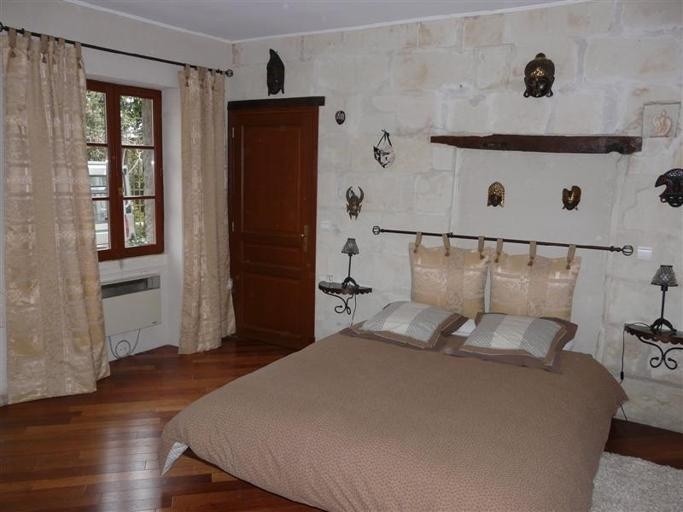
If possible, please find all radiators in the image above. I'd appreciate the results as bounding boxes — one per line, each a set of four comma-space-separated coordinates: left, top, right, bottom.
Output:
99, 273, 166, 339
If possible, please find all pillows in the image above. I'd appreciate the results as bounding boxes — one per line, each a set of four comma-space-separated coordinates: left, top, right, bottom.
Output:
487, 245, 579, 329
346, 295, 469, 353
410, 237, 487, 315
448, 313, 583, 374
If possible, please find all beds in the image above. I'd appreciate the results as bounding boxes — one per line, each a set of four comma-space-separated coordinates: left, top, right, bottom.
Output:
161, 226, 636, 512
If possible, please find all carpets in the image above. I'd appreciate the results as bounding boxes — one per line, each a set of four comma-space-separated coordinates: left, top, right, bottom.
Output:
588, 445, 683, 512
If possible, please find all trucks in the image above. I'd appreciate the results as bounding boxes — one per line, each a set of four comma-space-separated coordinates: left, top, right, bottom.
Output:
87, 161, 134, 251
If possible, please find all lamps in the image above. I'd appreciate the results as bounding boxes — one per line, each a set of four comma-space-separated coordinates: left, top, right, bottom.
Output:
646, 262, 679, 333
338, 236, 361, 292
